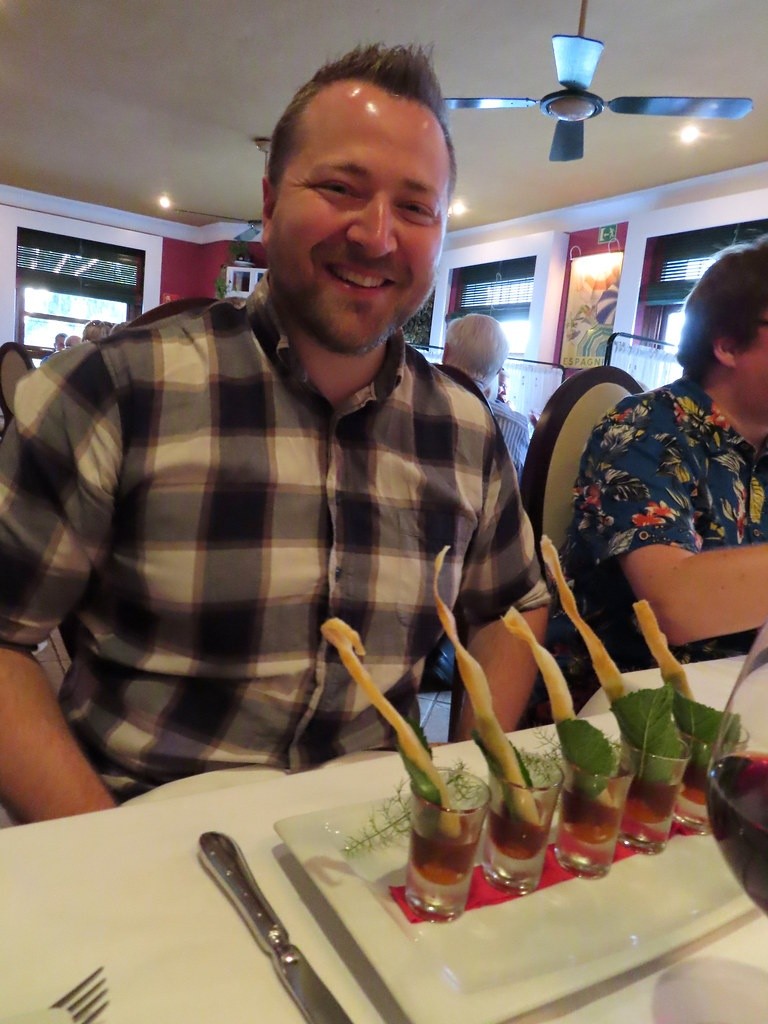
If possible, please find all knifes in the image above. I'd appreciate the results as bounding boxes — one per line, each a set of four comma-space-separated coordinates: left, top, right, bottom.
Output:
197, 831, 353, 1024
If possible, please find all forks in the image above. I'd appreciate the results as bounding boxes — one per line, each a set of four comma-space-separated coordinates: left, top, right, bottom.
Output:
51, 966, 109, 1024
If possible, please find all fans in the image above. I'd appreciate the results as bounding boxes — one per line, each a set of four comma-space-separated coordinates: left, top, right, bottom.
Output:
176, 137, 272, 241
441, 0, 755, 161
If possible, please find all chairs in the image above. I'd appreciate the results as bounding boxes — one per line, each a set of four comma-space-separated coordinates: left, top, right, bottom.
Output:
521, 367, 642, 577
0, 336, 45, 426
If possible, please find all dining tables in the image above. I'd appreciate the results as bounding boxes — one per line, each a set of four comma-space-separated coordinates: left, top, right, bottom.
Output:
0, 654, 768, 1024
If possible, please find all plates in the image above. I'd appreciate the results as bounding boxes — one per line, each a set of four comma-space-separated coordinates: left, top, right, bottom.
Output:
275, 781, 755, 1022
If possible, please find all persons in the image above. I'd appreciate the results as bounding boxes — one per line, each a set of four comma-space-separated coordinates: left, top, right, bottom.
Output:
514, 232, 768, 727
39, 320, 129, 366
0, 36, 553, 823
441, 313, 530, 483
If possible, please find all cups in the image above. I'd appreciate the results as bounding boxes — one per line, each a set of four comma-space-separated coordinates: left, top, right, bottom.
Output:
618, 732, 690, 856
482, 753, 565, 894
704, 617, 768, 914
406, 768, 492, 922
674, 724, 752, 830
556, 741, 636, 878
